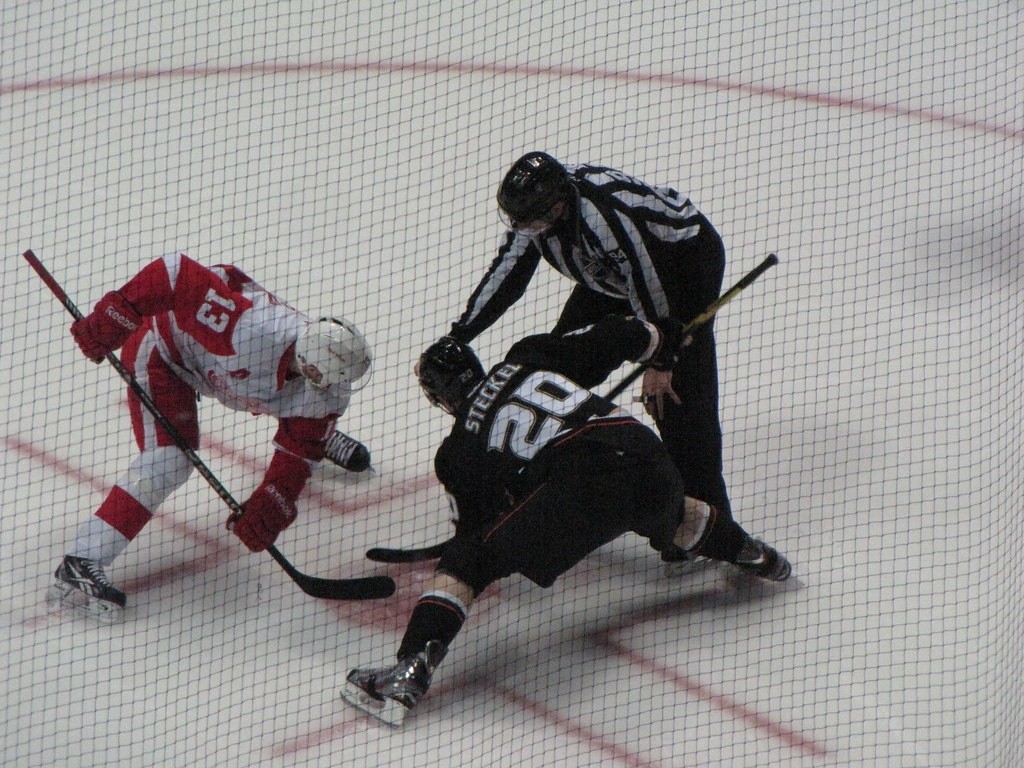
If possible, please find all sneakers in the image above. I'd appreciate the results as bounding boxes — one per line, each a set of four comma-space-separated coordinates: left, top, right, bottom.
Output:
324, 431, 375, 474
46, 552, 126, 624
340, 640, 447, 726
732, 539, 792, 582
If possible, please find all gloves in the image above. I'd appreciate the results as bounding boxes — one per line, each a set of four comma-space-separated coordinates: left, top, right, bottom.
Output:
649, 317, 692, 371
70, 290, 143, 364
225, 480, 297, 553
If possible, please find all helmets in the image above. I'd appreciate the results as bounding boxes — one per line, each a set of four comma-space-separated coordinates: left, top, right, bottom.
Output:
415, 335, 485, 416
295, 317, 373, 388
499, 151, 570, 222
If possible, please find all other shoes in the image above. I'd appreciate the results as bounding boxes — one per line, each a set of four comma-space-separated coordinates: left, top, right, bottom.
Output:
662, 549, 711, 574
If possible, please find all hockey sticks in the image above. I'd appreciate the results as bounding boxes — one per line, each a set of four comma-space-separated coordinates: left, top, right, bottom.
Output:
362, 250, 782, 566
22, 247, 398, 606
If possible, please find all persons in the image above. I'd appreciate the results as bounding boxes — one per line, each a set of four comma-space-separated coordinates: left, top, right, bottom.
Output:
340, 314, 809, 726
416, 152, 734, 562
43, 251, 374, 624
